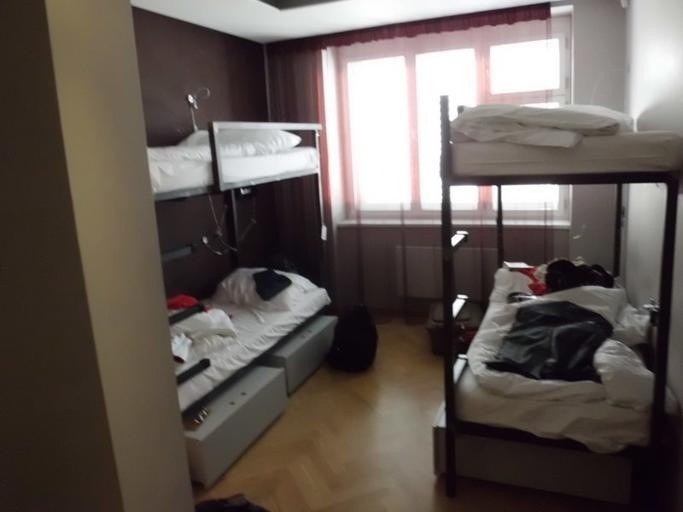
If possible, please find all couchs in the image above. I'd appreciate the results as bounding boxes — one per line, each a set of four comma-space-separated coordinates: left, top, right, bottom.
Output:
145, 121, 334, 418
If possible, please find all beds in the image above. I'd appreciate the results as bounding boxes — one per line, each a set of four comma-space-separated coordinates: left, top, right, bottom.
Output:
440, 95, 681, 511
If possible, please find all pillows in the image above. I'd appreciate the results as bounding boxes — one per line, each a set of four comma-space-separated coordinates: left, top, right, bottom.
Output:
179, 125, 301, 149
214, 267, 319, 310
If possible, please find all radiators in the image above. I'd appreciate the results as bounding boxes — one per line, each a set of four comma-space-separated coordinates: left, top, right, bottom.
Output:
397, 244, 499, 302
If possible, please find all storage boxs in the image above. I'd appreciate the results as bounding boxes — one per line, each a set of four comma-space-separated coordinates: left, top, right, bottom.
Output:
264, 316, 339, 396
433, 398, 632, 505
183, 365, 287, 491
